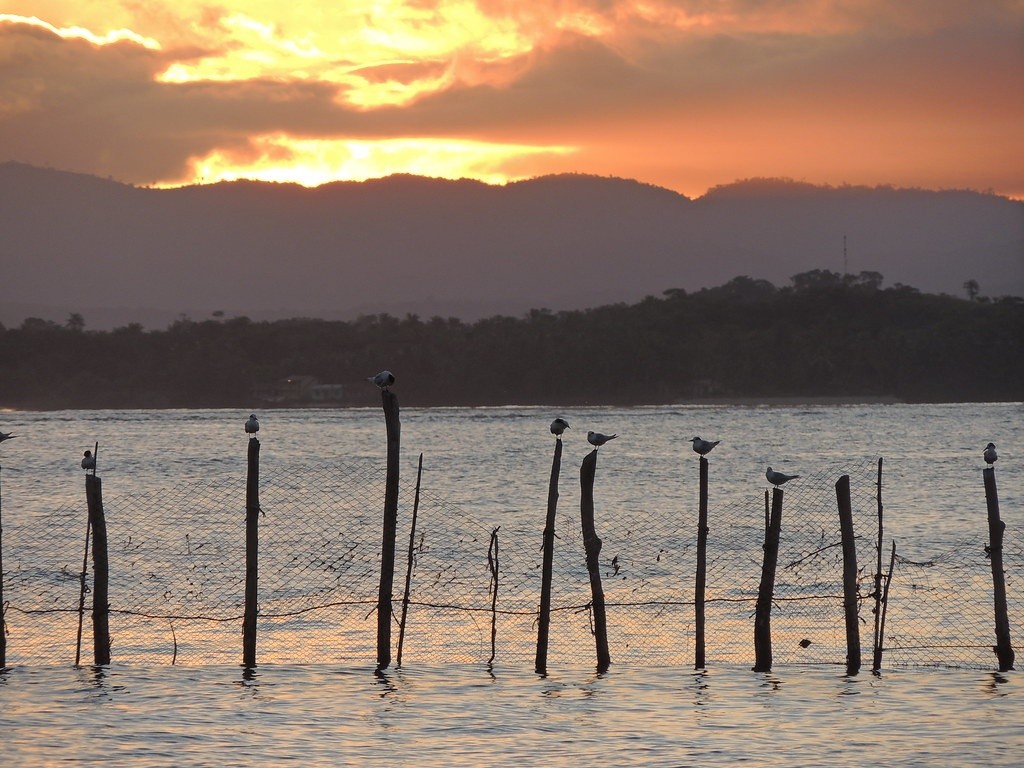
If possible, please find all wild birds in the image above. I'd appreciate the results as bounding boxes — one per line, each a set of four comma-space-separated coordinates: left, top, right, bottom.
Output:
587, 431, 619, 451
81, 450, 95, 475
982, 443, 998, 468
689, 437, 721, 458
549, 418, 571, 439
244, 414, 259, 439
766, 467, 800, 488
367, 370, 396, 393
